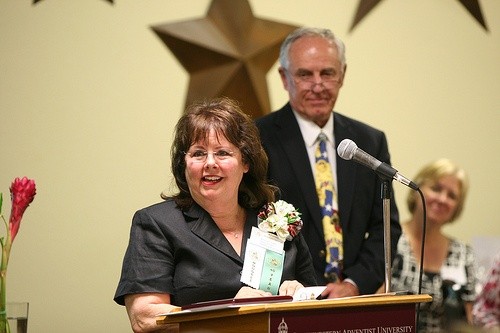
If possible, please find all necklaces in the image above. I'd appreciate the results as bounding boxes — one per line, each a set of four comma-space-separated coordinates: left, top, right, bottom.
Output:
222, 226, 243, 238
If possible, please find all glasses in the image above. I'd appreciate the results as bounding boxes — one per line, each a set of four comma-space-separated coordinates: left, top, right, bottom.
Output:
185, 147, 241, 162
284, 67, 344, 89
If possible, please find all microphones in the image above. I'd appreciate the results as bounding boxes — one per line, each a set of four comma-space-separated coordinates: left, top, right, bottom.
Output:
337, 139, 419, 191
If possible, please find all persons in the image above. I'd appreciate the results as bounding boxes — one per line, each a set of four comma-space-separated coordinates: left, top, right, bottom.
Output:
113, 96, 319, 333
252, 26, 403, 299
390, 159, 500, 333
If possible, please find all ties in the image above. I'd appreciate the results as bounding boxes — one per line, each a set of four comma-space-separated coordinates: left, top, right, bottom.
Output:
313, 131, 345, 280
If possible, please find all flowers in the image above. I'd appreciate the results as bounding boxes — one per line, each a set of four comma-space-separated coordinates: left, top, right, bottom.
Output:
0, 177, 37, 333
259, 200, 305, 242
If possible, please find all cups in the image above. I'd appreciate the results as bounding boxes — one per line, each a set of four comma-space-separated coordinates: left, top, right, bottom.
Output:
6, 302, 28, 333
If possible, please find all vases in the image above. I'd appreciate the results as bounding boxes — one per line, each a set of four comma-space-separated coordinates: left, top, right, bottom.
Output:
0, 302, 29, 333
